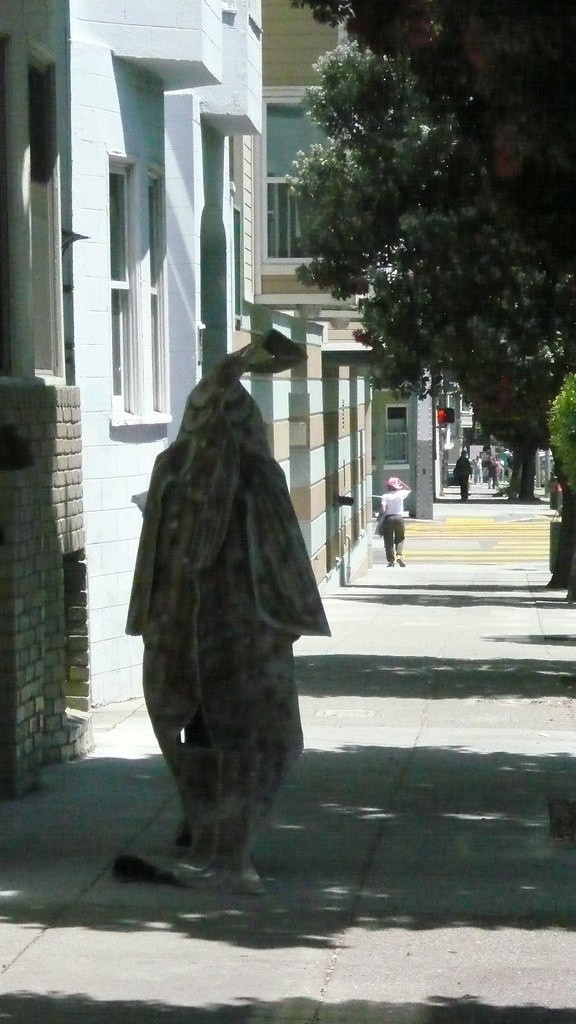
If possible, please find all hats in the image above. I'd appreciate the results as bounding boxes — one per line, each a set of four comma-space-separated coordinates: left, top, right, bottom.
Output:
385, 477, 403, 489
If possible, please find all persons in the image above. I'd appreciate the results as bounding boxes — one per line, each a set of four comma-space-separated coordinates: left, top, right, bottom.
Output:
455, 450, 471, 504
473, 453, 504, 489
379, 477, 413, 568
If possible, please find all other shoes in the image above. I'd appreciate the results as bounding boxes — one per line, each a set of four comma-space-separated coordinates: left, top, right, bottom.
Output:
387, 562, 394, 567
396, 556, 405, 568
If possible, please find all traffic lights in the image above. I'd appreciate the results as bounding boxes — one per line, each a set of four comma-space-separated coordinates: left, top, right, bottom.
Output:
437, 407, 454, 424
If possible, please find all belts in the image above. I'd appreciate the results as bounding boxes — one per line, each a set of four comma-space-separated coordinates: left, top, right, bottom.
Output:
385, 514, 395, 519
